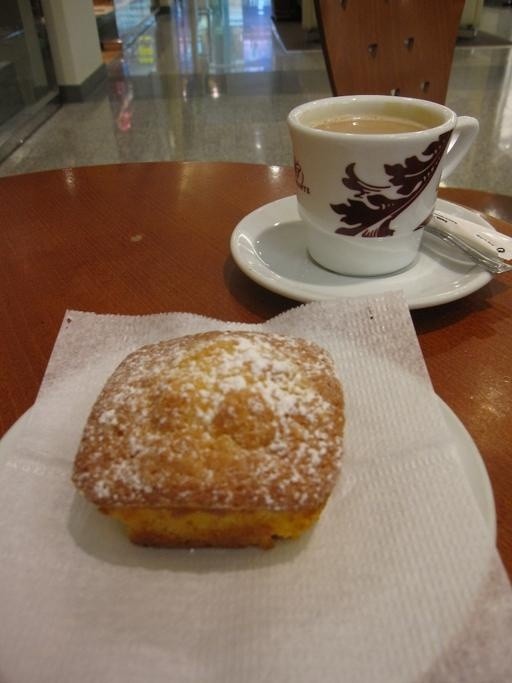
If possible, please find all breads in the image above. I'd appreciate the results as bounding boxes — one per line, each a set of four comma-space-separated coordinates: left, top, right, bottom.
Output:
67, 326, 348, 551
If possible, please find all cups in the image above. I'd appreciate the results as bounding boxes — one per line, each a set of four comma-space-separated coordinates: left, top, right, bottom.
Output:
284, 95, 479, 277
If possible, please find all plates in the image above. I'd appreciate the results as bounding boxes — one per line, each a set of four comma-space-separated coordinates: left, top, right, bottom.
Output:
0, 342, 497, 683
230, 194, 504, 310
